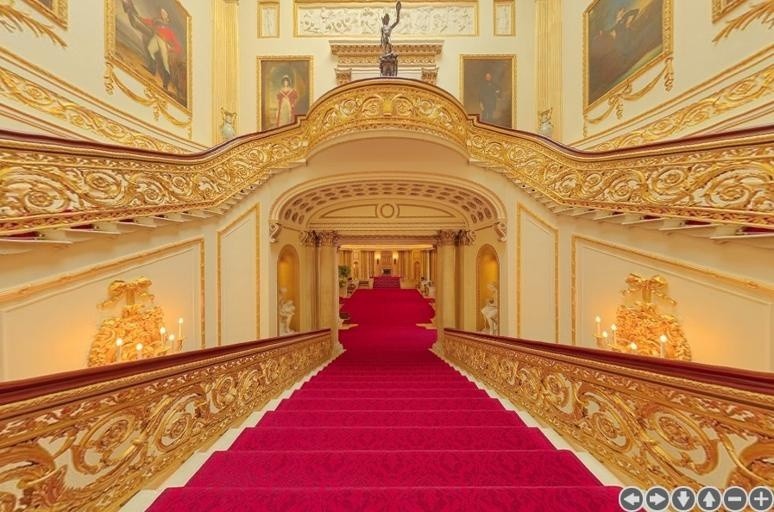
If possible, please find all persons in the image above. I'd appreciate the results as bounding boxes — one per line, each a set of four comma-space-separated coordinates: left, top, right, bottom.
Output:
480, 70, 501, 124
276, 73, 299, 128
379, 1, 402, 53
139, 8, 182, 90
598, 7, 639, 48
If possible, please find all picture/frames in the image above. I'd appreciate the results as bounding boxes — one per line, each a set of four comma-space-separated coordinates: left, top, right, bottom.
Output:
581, 1, 674, 118
459, 51, 518, 133
255, 55, 313, 133
104, 0, 195, 116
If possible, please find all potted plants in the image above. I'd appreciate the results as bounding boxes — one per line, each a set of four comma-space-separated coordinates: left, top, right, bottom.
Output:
338, 264, 352, 288
